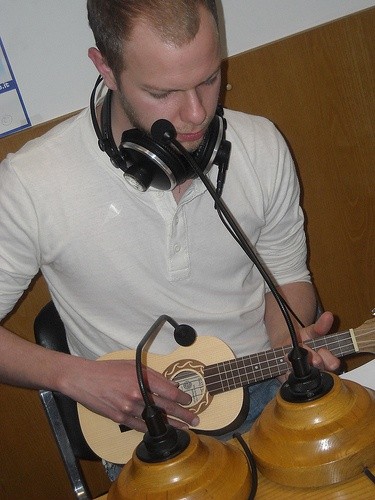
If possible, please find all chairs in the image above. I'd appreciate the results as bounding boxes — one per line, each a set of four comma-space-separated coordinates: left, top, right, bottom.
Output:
32, 281, 348, 500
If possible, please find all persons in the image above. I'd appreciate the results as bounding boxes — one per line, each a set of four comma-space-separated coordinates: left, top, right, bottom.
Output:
0, 2, 341, 481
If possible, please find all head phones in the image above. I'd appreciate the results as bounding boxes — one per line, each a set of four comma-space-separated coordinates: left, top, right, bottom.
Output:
90, 73, 228, 193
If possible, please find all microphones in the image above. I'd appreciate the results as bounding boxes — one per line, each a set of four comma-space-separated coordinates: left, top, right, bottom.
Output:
150, 118, 334, 404
135, 314, 197, 462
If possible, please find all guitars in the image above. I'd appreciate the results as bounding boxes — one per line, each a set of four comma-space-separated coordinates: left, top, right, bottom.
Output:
76, 307, 375, 465
